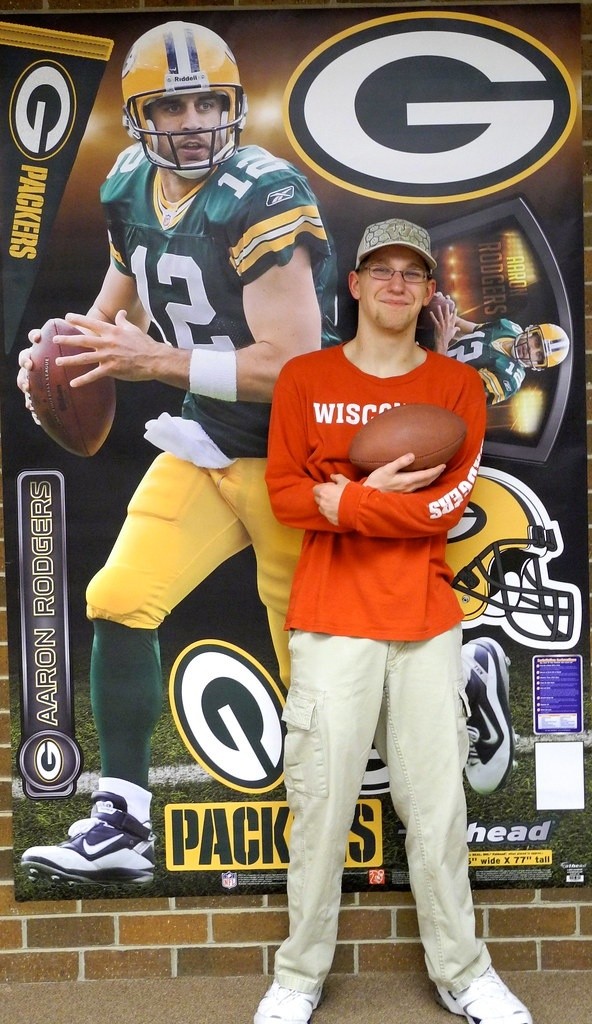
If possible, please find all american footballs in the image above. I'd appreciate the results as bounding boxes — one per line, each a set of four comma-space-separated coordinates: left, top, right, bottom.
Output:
349, 400, 467, 479
416, 295, 457, 331
28, 318, 117, 459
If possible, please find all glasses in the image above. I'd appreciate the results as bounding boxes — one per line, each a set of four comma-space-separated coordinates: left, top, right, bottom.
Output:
359, 264, 433, 283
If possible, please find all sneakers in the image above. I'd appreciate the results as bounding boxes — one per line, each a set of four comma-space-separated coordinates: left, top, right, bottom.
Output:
20, 791, 157, 890
461, 636, 521, 795
435, 964, 533, 1024
254, 979, 322, 1024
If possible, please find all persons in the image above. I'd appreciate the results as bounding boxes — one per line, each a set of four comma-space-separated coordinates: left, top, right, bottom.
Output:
252, 216, 537, 1024
18, 21, 514, 887
430, 292, 571, 406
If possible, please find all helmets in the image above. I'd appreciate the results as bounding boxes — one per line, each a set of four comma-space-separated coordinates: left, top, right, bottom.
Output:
514, 323, 570, 371
121, 20, 249, 179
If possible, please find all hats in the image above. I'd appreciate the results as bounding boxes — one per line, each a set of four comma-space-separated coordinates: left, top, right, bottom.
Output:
355, 218, 438, 274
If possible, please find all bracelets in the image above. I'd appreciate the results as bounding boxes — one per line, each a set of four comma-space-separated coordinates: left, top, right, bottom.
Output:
188, 348, 239, 403
